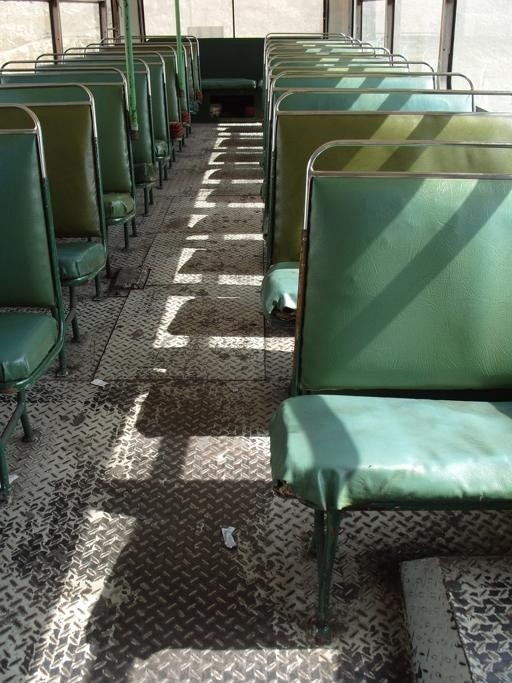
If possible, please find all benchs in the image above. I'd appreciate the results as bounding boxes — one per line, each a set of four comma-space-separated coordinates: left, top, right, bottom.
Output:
252, 28, 511, 631
1, 34, 204, 501
145, 37, 266, 124
